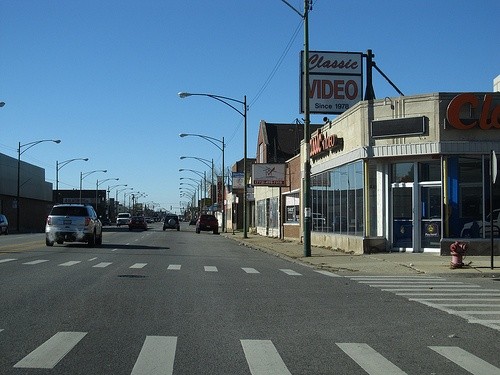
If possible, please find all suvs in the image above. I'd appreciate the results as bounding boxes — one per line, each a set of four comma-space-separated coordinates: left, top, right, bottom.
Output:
162, 214, 181, 231
115, 212, 133, 227
195, 214, 219, 234
44, 203, 103, 247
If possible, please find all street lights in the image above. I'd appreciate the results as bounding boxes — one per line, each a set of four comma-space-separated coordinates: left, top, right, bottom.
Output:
56, 157, 89, 205
16, 139, 61, 233
95, 177, 120, 212
178, 91, 248, 239
179, 182, 199, 217
179, 132, 225, 232
108, 184, 128, 218
179, 177, 203, 215
79, 169, 108, 204
179, 188, 197, 219
178, 168, 207, 214
115, 187, 161, 223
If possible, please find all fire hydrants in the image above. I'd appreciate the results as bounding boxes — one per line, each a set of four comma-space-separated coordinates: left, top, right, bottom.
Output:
449, 241, 469, 270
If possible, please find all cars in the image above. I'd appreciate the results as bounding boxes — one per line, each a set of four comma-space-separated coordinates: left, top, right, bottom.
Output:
0, 214, 9, 235
128, 216, 148, 231
189, 219, 197, 225
98, 214, 112, 226
459, 209, 500, 238
